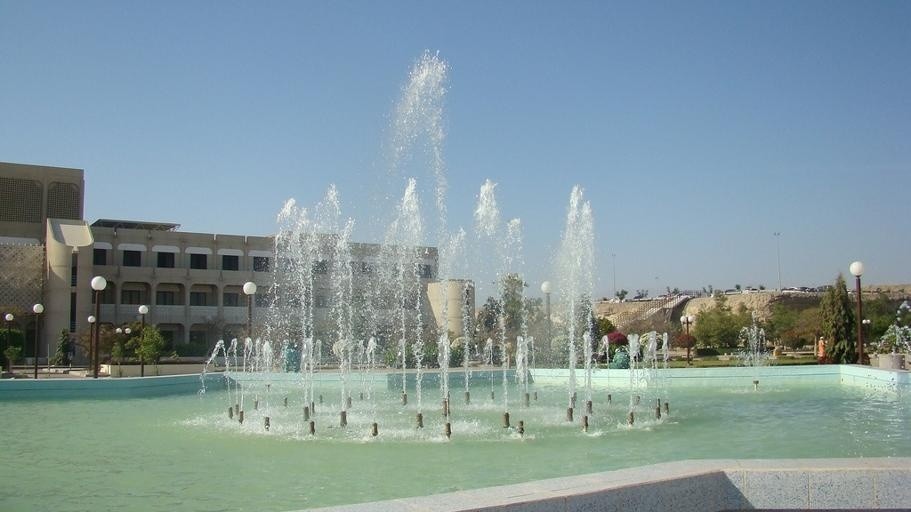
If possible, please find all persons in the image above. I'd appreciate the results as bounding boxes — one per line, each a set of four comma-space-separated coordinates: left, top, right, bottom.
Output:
818, 336, 828, 359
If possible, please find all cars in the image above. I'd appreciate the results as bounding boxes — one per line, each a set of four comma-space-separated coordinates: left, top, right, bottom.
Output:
607, 294, 695, 303
725, 285, 834, 294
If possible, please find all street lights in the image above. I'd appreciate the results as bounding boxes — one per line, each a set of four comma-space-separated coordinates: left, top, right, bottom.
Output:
541, 281, 554, 350
773, 232, 782, 288
680, 315, 693, 363
33, 304, 45, 379
242, 281, 257, 338
611, 253, 616, 296
139, 305, 149, 377
88, 275, 107, 378
850, 261, 864, 364
5, 314, 14, 346
115, 327, 132, 336
